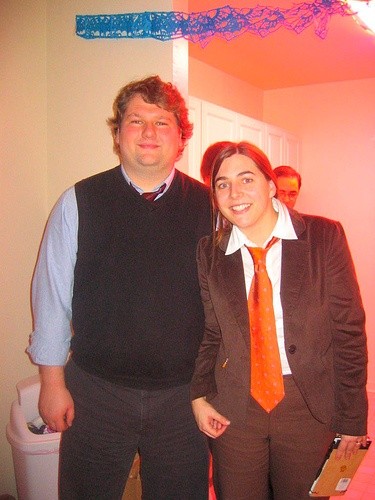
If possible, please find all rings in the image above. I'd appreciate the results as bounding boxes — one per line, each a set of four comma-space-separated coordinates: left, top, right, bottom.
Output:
356, 441, 362, 445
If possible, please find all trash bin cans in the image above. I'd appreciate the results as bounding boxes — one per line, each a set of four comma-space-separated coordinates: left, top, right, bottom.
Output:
6, 374, 64, 499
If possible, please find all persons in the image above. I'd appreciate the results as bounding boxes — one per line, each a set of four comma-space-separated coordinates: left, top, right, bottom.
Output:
273, 166, 301, 209
200, 141, 235, 187
25, 74, 220, 500
191, 139, 368, 500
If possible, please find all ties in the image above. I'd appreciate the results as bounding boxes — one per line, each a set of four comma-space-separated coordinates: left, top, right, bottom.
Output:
243, 236, 286, 414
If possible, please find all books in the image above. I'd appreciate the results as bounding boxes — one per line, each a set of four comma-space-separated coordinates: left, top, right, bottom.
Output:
309, 436, 372, 497
